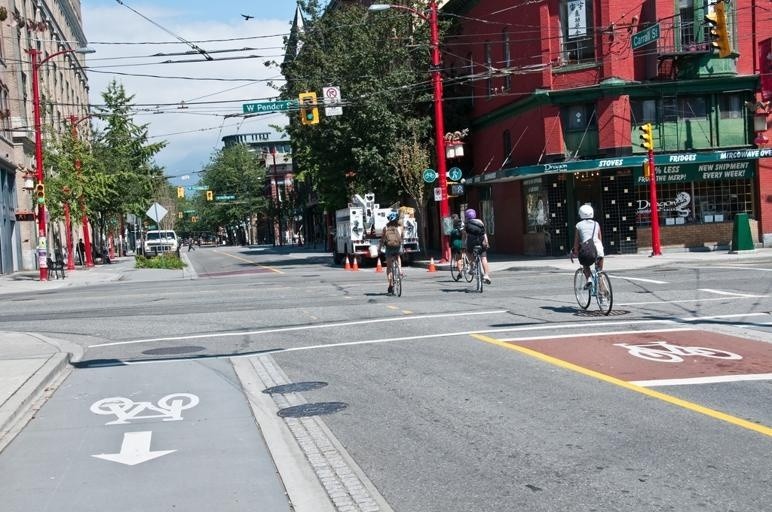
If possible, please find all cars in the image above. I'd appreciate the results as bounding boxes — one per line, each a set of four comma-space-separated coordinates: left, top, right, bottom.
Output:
280, 227, 303, 244
182, 230, 217, 247
656, 191, 756, 225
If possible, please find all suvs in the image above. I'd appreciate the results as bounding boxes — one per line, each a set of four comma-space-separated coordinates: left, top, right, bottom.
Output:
143, 229, 181, 261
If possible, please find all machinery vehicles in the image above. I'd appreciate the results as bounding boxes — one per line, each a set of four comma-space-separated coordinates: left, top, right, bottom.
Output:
332, 190, 421, 268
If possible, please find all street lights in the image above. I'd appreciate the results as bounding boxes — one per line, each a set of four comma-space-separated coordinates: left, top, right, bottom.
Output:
31, 46, 96, 281
71, 111, 138, 268
368, 4, 452, 261
247, 148, 282, 246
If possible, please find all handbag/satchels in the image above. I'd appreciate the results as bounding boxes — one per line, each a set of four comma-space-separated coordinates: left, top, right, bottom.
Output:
465, 220, 486, 236
578, 239, 598, 267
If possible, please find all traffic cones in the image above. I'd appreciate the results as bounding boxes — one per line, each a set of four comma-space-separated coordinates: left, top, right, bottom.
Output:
427, 256, 438, 272
374, 257, 385, 272
343, 256, 353, 271
350, 257, 360, 272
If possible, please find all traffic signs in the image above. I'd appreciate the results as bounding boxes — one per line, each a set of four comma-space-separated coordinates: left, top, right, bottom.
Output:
183, 210, 195, 213
215, 195, 236, 201
629, 23, 661, 49
243, 100, 299, 114
188, 185, 209, 190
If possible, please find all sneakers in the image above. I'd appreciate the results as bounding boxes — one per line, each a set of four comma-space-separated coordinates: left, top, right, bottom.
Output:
583, 280, 593, 290
388, 286, 394, 294
456, 256, 492, 285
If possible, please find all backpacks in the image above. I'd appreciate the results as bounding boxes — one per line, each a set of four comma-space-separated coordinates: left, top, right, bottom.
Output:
382, 225, 403, 248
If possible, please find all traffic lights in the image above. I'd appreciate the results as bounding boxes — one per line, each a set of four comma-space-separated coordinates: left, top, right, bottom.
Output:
207, 191, 212, 201
303, 97, 314, 121
448, 184, 465, 196
36, 184, 46, 205
704, 3, 730, 59
638, 123, 652, 150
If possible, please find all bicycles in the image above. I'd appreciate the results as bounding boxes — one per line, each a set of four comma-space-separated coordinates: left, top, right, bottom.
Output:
570, 247, 613, 315
447, 236, 474, 283
462, 245, 487, 293
379, 250, 404, 297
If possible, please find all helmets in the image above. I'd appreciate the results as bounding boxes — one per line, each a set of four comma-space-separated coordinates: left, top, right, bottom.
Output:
465, 208, 477, 219
578, 204, 594, 219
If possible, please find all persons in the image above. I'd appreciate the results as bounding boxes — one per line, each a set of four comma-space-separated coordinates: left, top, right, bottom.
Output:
378, 212, 404, 292
186, 236, 195, 252
450, 220, 466, 278
573, 205, 604, 302
462, 209, 491, 284
76, 239, 85, 265
